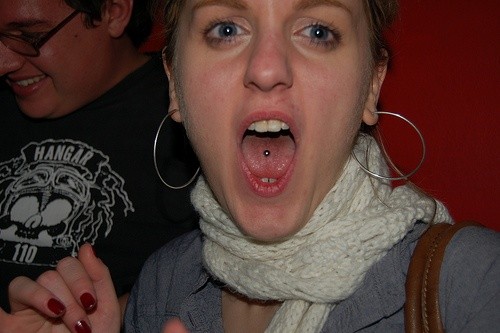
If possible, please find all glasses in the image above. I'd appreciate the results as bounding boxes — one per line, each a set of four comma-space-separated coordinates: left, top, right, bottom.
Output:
0, 8, 82, 58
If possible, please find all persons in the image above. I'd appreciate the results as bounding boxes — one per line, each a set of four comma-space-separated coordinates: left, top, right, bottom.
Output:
1, 1, 499, 331
1, 0, 210, 316
